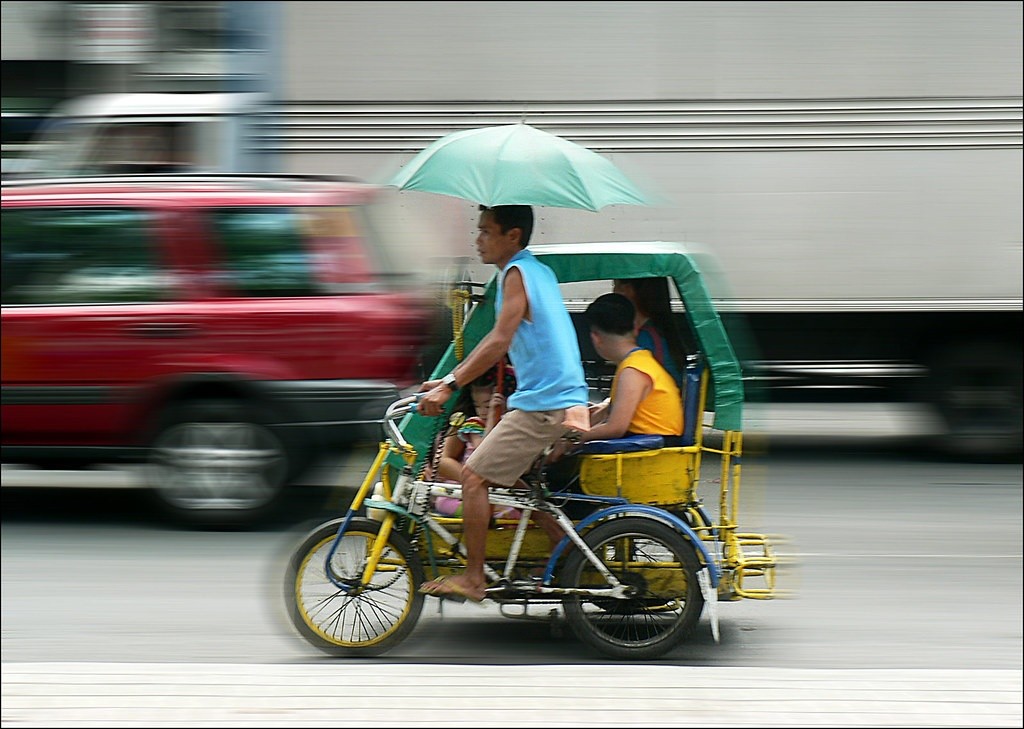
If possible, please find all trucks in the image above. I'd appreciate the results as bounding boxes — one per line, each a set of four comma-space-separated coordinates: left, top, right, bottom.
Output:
40, 0, 1024, 423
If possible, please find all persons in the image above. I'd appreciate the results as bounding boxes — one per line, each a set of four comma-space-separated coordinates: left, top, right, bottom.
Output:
416, 204, 590, 609
410, 203, 684, 530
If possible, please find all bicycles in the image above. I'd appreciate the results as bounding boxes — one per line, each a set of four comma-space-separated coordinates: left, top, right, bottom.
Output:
283, 392, 719, 661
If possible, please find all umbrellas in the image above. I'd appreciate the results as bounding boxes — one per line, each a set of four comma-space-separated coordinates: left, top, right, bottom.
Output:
391, 116, 656, 212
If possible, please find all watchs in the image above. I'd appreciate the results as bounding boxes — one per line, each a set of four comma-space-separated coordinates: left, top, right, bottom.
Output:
444, 373, 460, 391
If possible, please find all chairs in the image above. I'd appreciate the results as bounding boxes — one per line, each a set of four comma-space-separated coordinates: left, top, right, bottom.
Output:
573, 365, 701, 499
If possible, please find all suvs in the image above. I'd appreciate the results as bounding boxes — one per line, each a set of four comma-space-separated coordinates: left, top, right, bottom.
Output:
0, 174, 440, 530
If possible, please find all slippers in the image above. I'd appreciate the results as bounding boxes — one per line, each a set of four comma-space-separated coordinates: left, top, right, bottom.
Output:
417, 574, 489, 607
